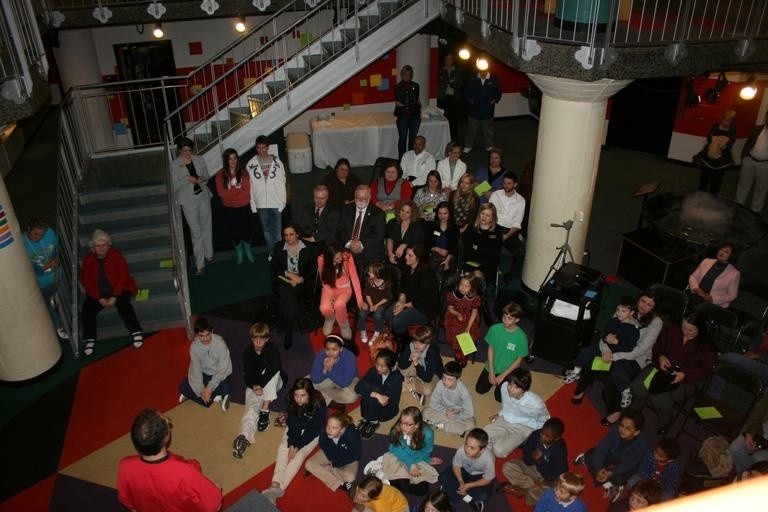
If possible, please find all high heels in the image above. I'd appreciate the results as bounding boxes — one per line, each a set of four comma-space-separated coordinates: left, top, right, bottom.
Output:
571, 390, 585, 406
600, 417, 615, 427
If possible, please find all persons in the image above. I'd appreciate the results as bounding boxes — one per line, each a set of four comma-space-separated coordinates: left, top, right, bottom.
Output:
694, 110, 735, 194
448, 173, 479, 232
534, 471, 587, 512
501, 417, 569, 507
400, 136, 437, 194
443, 275, 481, 369
418, 490, 454, 512
563, 297, 634, 408
393, 65, 422, 163
605, 478, 662, 512
626, 436, 684, 502
385, 242, 438, 341
425, 201, 457, 288
337, 184, 386, 261
362, 406, 443, 484
413, 169, 448, 221
437, 54, 462, 141
357, 261, 391, 346
574, 408, 646, 504
572, 290, 663, 428
731, 422, 765, 471
354, 348, 405, 441
245, 135, 287, 264
351, 476, 410, 512
169, 137, 214, 276
269, 225, 313, 350
459, 204, 503, 258
688, 241, 740, 308
437, 140, 467, 190
178, 316, 233, 411
368, 160, 413, 211
117, 408, 224, 512
482, 367, 551, 458
304, 412, 362, 500
261, 377, 328, 506
476, 301, 528, 403
21, 217, 70, 339
490, 171, 526, 285
630, 310, 715, 436
304, 333, 360, 408
463, 69, 502, 154
475, 148, 508, 203
322, 157, 363, 210
421, 361, 475, 438
384, 201, 418, 263
398, 326, 443, 407
316, 241, 368, 339
438, 428, 495, 512
215, 148, 255, 265
79, 229, 145, 356
734, 113, 767, 213
232, 321, 284, 459
295, 184, 339, 249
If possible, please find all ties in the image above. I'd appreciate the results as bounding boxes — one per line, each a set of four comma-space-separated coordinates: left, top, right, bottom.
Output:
314, 209, 320, 233
351, 211, 362, 241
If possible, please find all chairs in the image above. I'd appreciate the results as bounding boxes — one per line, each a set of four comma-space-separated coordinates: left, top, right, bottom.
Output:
677, 359, 765, 459
310, 262, 360, 328
423, 269, 443, 346
586, 283, 688, 401
665, 303, 738, 418
370, 156, 400, 185
361, 264, 402, 332
436, 274, 486, 365
709, 289, 768, 352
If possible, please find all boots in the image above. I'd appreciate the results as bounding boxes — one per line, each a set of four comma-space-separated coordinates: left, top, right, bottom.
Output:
242, 240, 255, 263
233, 241, 243, 265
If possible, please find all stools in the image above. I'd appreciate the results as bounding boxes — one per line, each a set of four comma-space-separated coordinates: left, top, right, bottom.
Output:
535, 263, 606, 356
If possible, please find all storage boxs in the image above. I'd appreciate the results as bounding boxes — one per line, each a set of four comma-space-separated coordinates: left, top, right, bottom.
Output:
285, 132, 312, 174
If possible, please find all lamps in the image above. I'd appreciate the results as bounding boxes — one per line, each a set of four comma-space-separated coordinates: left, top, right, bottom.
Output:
438, 25, 456, 46
233, 17, 248, 34
681, 81, 702, 108
740, 76, 759, 102
151, 24, 165, 40
704, 77, 729, 102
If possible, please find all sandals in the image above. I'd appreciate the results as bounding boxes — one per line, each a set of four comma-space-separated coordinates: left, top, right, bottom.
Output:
131, 332, 144, 348
83, 339, 95, 356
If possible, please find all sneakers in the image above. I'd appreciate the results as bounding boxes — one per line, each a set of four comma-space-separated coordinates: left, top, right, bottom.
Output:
57, 328, 69, 339
562, 371, 580, 384
322, 318, 335, 335
262, 487, 285, 498
620, 391, 632, 408
179, 393, 189, 403
604, 484, 624, 505
368, 334, 377, 346
575, 453, 585, 465
473, 499, 484, 512
362, 420, 380, 440
219, 393, 230, 412
232, 435, 250, 459
424, 419, 437, 430
257, 409, 270, 432
208, 257, 216, 264
337, 320, 351, 340
411, 389, 425, 406
357, 419, 366, 436
192, 269, 207, 276
360, 328, 368, 343
342, 480, 356, 498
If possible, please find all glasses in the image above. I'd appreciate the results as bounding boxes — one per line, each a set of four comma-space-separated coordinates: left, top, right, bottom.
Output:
399, 421, 415, 427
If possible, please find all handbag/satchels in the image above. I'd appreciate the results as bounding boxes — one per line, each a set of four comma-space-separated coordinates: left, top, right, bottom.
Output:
648, 365, 688, 415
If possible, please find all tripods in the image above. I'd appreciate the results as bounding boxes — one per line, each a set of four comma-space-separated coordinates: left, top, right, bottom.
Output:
536, 220, 574, 295
